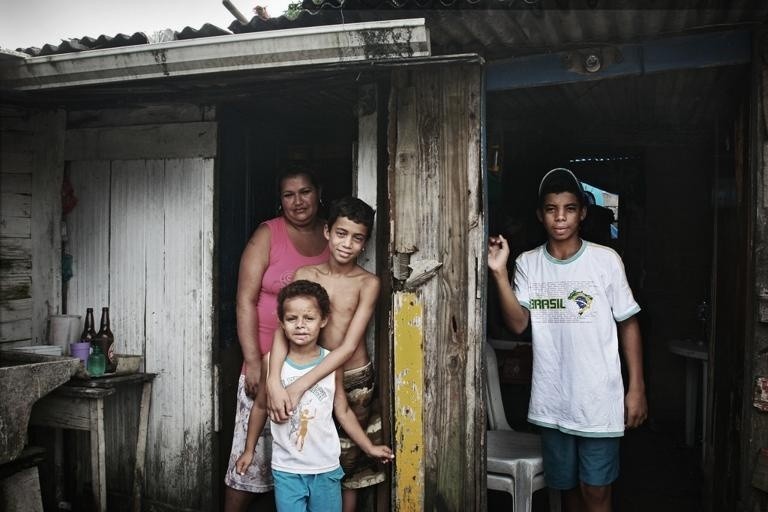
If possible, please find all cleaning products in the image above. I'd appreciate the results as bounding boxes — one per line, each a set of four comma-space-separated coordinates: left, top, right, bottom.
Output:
86, 341, 106, 375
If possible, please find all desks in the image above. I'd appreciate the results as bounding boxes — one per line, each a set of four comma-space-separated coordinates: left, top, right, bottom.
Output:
31, 370, 155, 512
666, 337, 710, 470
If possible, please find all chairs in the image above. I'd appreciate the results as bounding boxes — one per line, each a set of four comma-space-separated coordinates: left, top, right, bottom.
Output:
482, 339, 547, 512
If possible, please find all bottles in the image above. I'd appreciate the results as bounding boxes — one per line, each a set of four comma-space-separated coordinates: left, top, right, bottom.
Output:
80, 307, 116, 377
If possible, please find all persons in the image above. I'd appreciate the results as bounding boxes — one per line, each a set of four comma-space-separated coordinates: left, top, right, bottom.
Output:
220, 164, 332, 510
485, 161, 649, 511
234, 279, 395, 511
266, 195, 384, 511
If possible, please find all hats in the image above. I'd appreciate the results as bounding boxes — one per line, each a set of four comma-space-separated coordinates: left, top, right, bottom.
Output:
536, 166, 586, 204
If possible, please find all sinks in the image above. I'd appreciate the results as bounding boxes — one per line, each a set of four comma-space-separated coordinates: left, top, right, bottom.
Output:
1, 349, 82, 467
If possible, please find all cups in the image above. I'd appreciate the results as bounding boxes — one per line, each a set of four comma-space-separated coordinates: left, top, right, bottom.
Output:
69, 342, 90, 373
